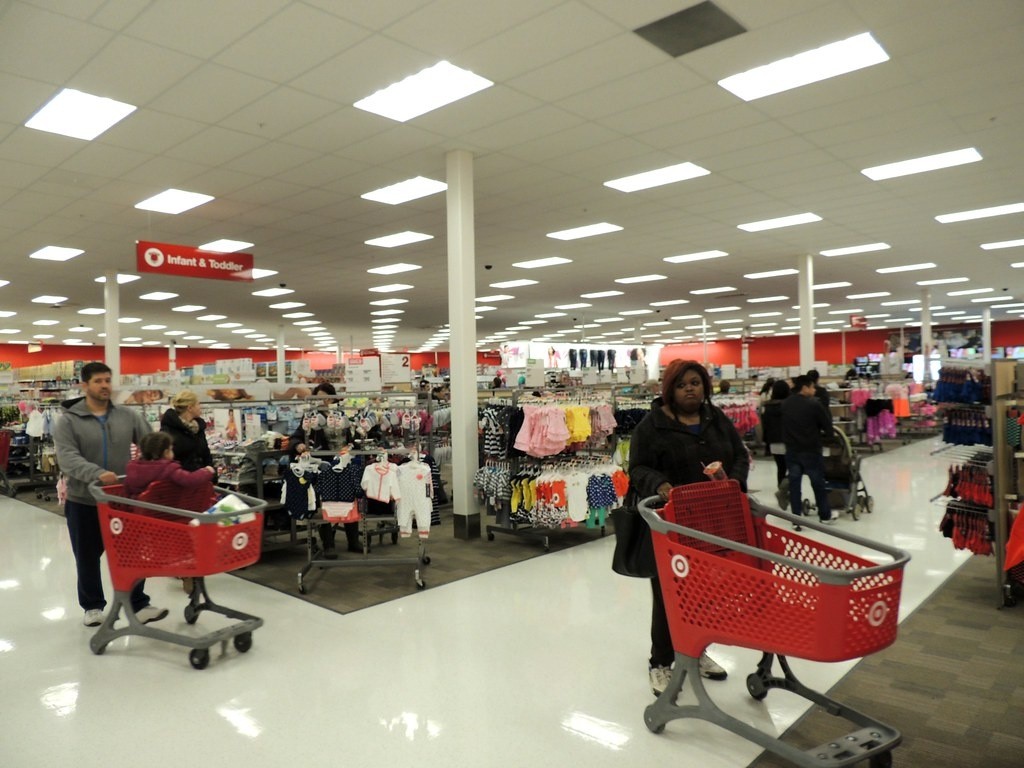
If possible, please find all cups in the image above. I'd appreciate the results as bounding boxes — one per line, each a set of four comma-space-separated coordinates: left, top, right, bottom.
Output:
703, 461, 729, 482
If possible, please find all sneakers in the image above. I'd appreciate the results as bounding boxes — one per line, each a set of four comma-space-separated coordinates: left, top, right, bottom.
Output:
698, 653, 726, 680
649, 665, 672, 696
84, 608, 103, 626
136, 606, 168, 624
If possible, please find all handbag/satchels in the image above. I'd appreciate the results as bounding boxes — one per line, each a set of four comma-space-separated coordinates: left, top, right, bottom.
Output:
611, 483, 657, 578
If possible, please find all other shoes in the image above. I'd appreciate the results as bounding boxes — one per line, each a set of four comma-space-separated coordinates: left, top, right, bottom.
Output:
324, 547, 338, 559
792, 522, 803, 530
348, 542, 370, 553
821, 510, 839, 523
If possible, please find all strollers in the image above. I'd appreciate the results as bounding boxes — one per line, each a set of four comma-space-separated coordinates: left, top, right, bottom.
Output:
803, 424, 875, 519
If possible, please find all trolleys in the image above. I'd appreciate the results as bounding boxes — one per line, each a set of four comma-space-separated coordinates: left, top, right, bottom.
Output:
88, 474, 268, 669
638, 475, 913, 768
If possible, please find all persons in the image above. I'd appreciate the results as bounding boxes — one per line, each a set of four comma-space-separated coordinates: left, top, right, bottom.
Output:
548, 346, 558, 368
612, 358, 750, 698
51, 362, 170, 626
492, 377, 501, 389
630, 349, 646, 361
759, 370, 839, 532
501, 345, 509, 366
418, 379, 450, 400
288, 383, 363, 553
159, 391, 218, 592
123, 431, 214, 500
225, 409, 237, 439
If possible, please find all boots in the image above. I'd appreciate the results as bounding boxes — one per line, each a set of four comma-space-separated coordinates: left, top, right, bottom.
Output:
775, 478, 790, 510
